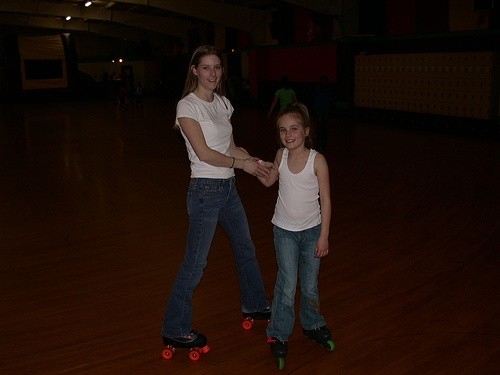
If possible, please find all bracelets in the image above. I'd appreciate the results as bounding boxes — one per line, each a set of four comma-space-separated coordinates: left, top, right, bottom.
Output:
257, 159, 265, 165
229, 156, 236, 169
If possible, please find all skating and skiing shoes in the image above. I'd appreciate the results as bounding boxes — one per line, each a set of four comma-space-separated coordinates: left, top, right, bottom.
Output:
303, 325, 334, 351
162, 333, 210, 360
242, 306, 272, 329
268, 337, 289, 369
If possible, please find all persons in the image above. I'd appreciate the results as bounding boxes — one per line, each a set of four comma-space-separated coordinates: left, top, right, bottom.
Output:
160, 44, 277, 360
268, 75, 298, 119
234, 102, 336, 369
